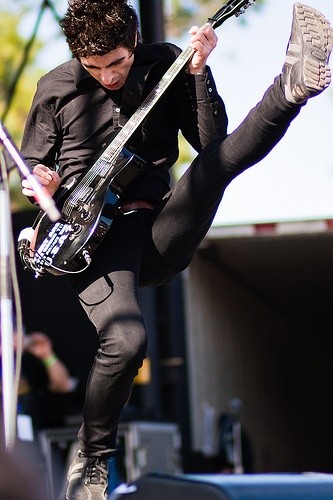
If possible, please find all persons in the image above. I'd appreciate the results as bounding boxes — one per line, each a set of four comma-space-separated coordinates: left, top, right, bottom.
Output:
18, 0, 333, 500
0, 325, 152, 500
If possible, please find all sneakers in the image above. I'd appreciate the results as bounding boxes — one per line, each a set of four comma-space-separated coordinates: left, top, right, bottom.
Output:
278, 0, 332, 104
67, 450, 111, 499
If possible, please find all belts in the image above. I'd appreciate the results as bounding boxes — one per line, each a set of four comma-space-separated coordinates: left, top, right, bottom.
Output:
117, 198, 161, 215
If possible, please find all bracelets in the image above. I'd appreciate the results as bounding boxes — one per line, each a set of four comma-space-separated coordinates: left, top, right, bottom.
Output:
42, 355, 57, 368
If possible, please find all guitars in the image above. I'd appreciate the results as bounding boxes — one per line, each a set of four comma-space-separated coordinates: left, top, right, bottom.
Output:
18, 0, 256, 279
